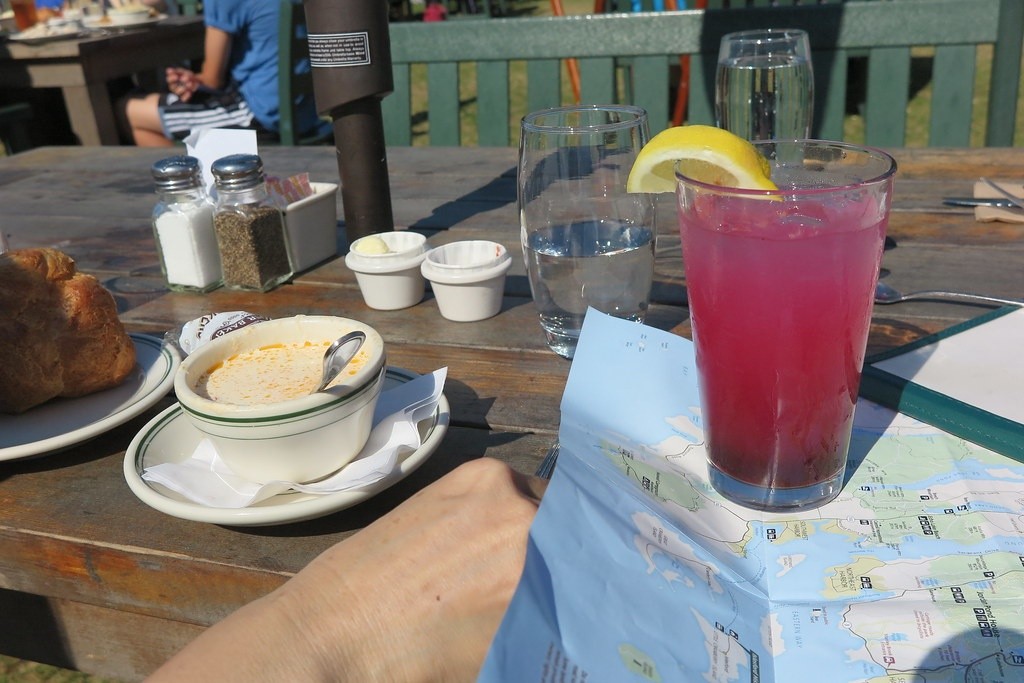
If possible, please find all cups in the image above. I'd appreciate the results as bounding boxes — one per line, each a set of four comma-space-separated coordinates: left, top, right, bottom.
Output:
714, 29, 815, 167
672, 137, 897, 513
515, 106, 658, 360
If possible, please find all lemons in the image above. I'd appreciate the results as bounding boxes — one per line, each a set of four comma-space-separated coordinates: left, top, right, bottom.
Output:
626, 125, 785, 202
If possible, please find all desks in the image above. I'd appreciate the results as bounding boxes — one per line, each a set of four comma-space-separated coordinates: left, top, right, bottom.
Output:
1, 147, 1024, 683
0, 16, 205, 156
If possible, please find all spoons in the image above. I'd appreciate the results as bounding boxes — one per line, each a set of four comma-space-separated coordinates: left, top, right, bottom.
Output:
873, 281, 1024, 309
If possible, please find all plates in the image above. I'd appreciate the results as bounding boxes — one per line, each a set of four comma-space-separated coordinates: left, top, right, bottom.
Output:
121, 364, 451, 529
84, 12, 170, 30
7, 28, 85, 44
0, 331, 182, 462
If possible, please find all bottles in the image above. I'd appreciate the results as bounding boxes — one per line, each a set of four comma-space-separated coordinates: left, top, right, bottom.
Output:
210, 153, 296, 293
148, 155, 225, 297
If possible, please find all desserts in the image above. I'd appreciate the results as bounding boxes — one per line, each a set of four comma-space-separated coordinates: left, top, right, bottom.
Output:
355, 236, 389, 255
193, 340, 364, 403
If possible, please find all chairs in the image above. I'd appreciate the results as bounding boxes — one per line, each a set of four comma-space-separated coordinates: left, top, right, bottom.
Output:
174, 0, 335, 146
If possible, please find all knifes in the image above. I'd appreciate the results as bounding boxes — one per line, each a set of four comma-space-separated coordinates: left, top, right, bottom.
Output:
942, 198, 1024, 209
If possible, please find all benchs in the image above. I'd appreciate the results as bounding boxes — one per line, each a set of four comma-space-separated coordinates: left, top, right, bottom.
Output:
381, 0, 1024, 149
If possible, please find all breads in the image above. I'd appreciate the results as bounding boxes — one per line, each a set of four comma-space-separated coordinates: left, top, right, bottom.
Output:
0, 246, 136, 415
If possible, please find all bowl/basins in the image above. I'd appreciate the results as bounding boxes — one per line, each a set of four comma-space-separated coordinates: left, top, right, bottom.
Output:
421, 240, 512, 323
278, 181, 339, 273
173, 315, 389, 494
345, 231, 430, 311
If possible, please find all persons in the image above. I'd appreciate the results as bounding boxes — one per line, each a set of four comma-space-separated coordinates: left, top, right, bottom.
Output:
115, 0, 319, 148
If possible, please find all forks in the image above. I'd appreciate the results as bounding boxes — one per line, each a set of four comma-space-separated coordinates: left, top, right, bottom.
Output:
980, 177, 1024, 209
